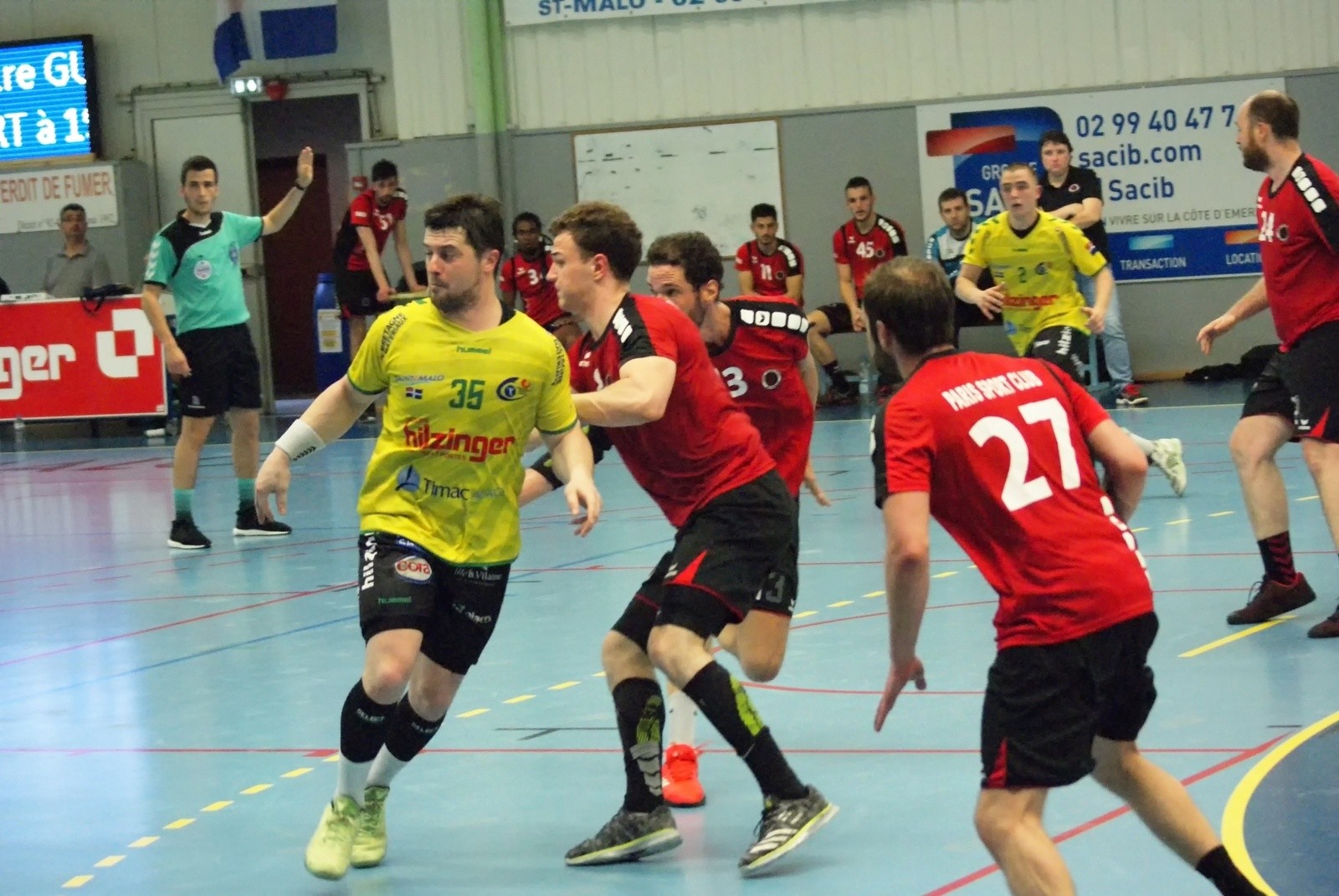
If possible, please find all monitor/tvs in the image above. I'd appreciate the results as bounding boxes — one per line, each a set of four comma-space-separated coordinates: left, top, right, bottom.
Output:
0, 34, 100, 174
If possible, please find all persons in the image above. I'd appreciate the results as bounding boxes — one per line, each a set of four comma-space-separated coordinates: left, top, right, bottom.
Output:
143, 144, 315, 549
43, 204, 114, 300
1198, 90, 1339, 640
251, 89, 1271, 896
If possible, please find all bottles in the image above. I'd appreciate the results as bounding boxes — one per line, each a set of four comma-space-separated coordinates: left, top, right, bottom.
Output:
858, 357, 871, 397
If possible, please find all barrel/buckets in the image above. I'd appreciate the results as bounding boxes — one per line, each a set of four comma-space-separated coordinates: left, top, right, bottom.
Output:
313, 272, 351, 388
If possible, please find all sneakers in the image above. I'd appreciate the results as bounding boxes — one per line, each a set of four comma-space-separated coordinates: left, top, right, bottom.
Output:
304, 794, 359, 879
662, 743, 706, 806
232, 507, 290, 535
1228, 573, 1316, 623
1148, 438, 1187, 497
351, 786, 392, 866
1116, 384, 1148, 404
1308, 606, 1339, 638
817, 388, 856, 405
565, 805, 681, 866
167, 521, 212, 548
873, 384, 893, 403
738, 783, 837, 872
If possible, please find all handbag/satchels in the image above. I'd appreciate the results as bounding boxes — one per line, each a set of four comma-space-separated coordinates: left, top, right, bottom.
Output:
80, 283, 134, 314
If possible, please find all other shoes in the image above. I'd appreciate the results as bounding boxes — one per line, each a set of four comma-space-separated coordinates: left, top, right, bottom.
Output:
359, 415, 376, 422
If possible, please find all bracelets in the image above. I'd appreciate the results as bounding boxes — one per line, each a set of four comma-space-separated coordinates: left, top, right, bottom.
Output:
294, 179, 307, 191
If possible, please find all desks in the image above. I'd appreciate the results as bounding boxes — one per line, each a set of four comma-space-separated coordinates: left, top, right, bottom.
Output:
0, 292, 176, 440
